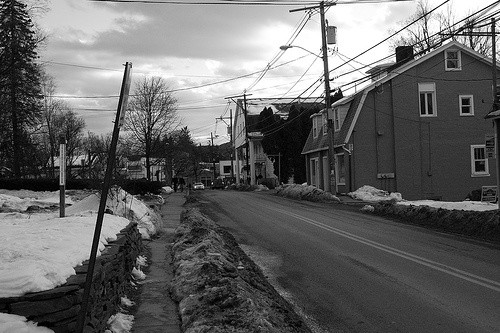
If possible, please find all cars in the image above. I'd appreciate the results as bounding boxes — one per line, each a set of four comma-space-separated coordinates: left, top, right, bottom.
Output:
193, 182, 205, 192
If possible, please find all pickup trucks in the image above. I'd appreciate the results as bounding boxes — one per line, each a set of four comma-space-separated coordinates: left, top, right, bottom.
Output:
210, 179, 226, 190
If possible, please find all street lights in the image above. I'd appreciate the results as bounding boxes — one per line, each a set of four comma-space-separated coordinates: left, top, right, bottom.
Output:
229, 95, 251, 188
279, 44, 338, 200
